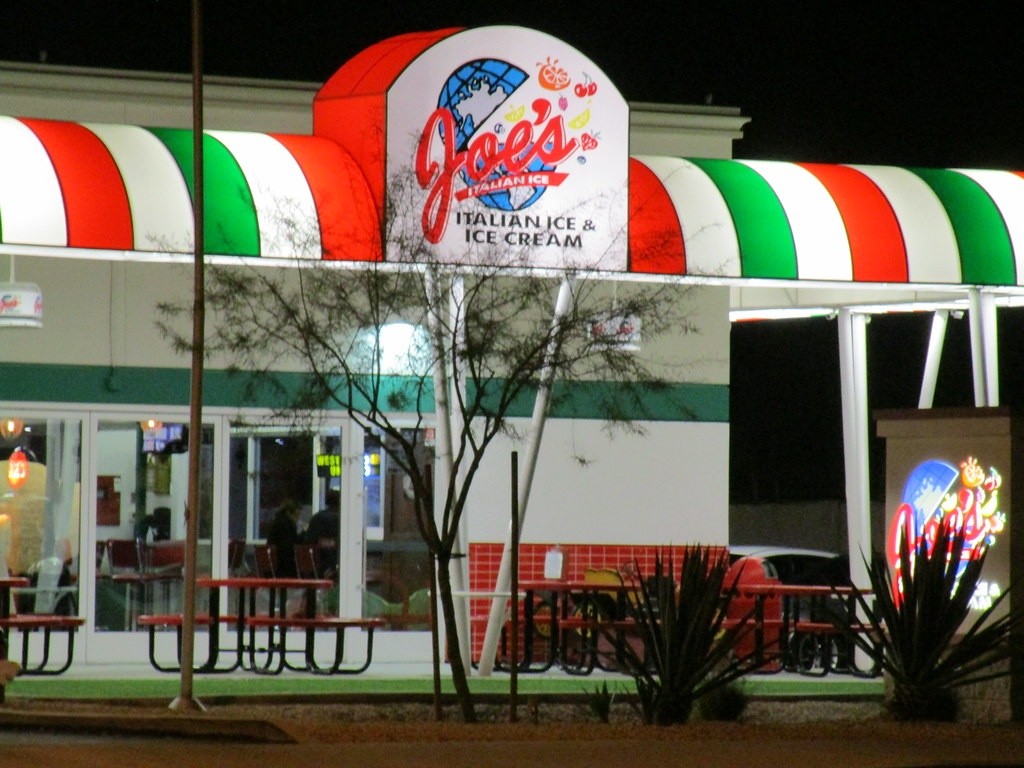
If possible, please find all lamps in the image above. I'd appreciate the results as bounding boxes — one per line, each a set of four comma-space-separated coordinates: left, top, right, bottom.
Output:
588, 280, 641, 354
0, 255, 42, 328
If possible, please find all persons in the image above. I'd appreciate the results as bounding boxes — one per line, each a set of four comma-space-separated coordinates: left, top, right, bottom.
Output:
307, 490, 341, 582
262, 500, 317, 617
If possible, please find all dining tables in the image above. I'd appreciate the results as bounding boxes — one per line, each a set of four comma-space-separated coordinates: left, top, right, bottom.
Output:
195, 579, 333, 676
0, 577, 27, 660
518, 579, 642, 676
721, 584, 874, 677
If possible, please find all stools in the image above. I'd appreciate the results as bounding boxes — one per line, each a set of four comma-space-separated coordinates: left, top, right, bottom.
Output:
138, 615, 385, 676
0, 615, 87, 676
470, 614, 661, 677
53, 537, 340, 631
713, 620, 888, 678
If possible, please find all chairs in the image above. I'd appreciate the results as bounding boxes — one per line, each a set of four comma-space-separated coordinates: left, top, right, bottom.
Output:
8, 568, 78, 616
